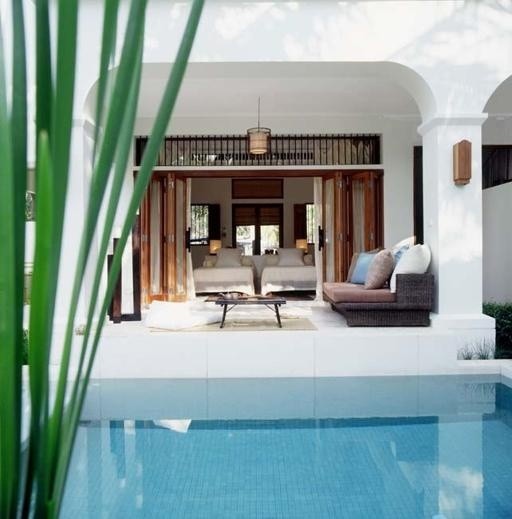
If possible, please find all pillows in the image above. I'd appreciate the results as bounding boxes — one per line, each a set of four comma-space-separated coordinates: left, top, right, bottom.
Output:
391, 234, 417, 269
389, 242, 432, 294
351, 252, 375, 284
364, 248, 394, 291
347, 251, 359, 283
214, 248, 244, 268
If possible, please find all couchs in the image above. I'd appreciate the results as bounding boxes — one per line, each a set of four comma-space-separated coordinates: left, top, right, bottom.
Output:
322, 271, 434, 328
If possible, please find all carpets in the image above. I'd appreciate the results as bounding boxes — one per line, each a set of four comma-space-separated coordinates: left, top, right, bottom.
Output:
144, 299, 319, 332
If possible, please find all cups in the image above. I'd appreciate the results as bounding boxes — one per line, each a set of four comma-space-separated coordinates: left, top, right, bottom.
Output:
231, 293, 241, 300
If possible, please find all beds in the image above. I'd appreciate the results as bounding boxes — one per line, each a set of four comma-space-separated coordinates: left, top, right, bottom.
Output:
194, 255, 258, 294
261, 253, 317, 296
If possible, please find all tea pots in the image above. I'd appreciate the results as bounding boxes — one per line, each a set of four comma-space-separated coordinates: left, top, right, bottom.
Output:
218, 290, 232, 299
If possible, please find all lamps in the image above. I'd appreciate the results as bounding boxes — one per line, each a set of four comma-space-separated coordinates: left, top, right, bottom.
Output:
275, 247, 307, 266
209, 239, 223, 256
245, 95, 272, 159
453, 139, 473, 185
296, 238, 308, 255
